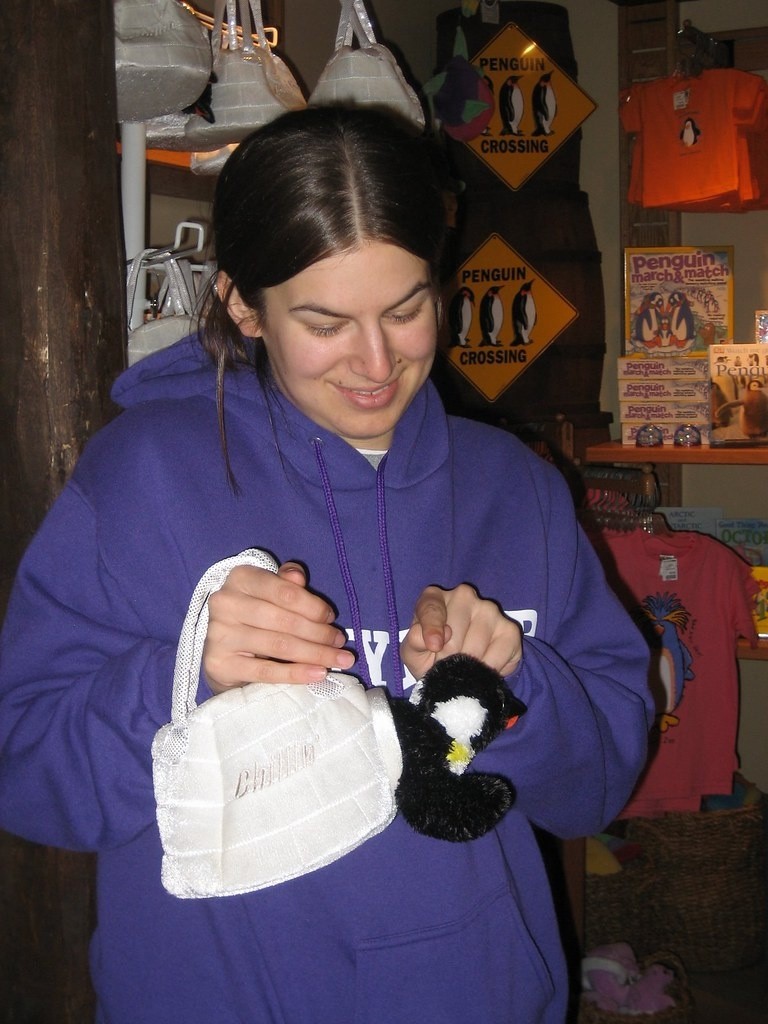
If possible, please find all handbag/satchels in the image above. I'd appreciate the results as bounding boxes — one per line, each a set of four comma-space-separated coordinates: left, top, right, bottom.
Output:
307, 0, 427, 126
114, 0, 306, 151
127, 246, 205, 367
150, 548, 403, 899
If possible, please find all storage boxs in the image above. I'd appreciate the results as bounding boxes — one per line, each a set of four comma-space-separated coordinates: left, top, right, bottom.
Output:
624, 246, 734, 359
617, 310, 768, 445
745, 566, 768, 638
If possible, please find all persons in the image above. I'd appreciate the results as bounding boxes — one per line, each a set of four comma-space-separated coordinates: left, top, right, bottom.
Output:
0, 101, 656, 1024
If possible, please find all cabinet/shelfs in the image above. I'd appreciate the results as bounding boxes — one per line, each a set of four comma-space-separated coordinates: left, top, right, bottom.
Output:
558, 0, 768, 950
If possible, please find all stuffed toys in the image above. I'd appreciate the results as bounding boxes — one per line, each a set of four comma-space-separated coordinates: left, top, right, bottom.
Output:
388, 652, 528, 843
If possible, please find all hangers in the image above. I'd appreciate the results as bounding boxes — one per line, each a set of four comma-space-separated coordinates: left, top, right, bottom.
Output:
569, 466, 671, 537
668, 23, 730, 84
